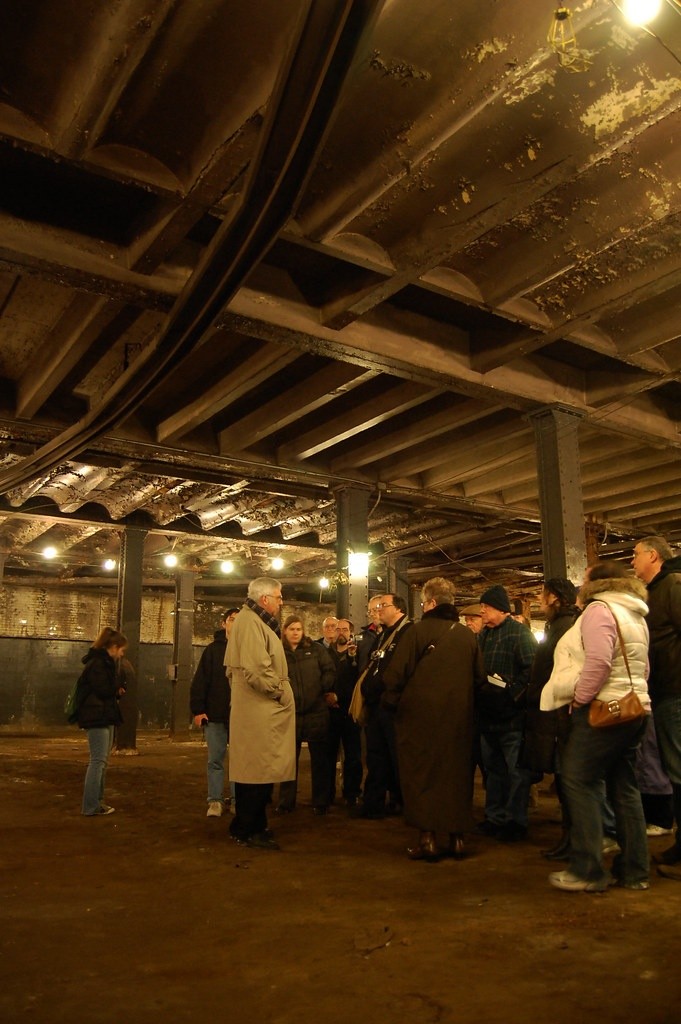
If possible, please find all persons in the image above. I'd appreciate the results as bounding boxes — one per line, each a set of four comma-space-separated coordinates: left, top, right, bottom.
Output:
190, 608, 238, 816
74, 626, 127, 815
282, 536, 681, 891
223, 576, 297, 851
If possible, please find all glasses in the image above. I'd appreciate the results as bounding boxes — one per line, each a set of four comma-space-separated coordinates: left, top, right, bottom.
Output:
632, 549, 650, 557
366, 608, 378, 615
376, 603, 395, 608
265, 594, 283, 602
420, 599, 431, 606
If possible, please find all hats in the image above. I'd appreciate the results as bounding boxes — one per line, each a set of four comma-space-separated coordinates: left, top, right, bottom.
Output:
479, 585, 511, 613
460, 604, 482, 617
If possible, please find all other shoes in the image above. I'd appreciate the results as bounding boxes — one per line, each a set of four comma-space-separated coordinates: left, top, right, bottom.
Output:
602, 838, 621, 854
80, 804, 115, 816
273, 803, 329, 816
207, 798, 279, 849
528, 784, 538, 807
548, 870, 607, 893
406, 831, 441, 862
646, 824, 673, 836
541, 840, 564, 860
450, 832, 464, 859
344, 797, 361, 807
387, 796, 403, 814
350, 796, 386, 819
652, 850, 681, 881
609, 873, 649, 890
475, 819, 528, 840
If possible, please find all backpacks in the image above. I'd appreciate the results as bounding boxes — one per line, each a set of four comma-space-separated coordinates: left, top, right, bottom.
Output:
64, 670, 94, 714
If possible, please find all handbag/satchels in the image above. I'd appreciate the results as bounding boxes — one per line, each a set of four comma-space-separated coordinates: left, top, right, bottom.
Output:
588, 690, 646, 728
